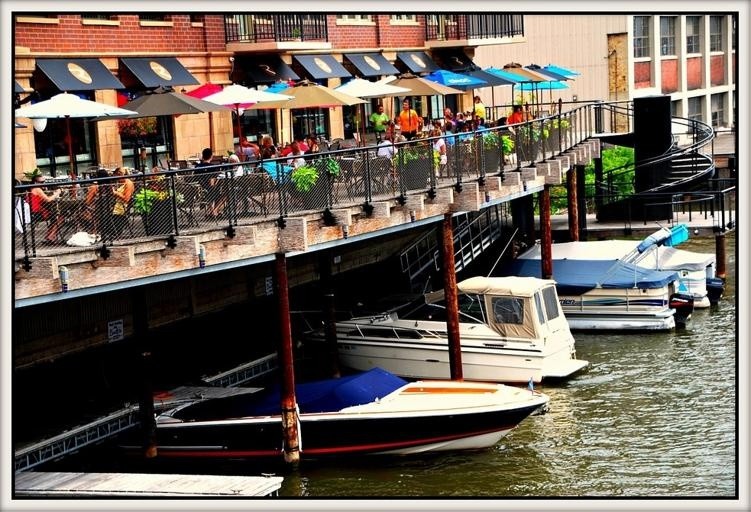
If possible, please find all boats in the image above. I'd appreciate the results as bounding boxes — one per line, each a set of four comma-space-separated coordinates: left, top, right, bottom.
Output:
310, 275, 589, 384
517, 238, 726, 308
502, 258, 693, 332
156, 367, 549, 461
13, 470, 283, 498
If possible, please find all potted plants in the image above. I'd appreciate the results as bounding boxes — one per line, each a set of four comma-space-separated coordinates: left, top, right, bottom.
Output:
515, 127, 541, 160
544, 119, 570, 150
470, 132, 514, 170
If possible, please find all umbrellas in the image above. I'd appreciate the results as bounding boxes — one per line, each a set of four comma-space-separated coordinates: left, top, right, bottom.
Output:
15, 92, 138, 179
92, 85, 229, 170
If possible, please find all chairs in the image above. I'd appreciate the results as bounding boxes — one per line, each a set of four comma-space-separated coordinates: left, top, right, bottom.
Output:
15, 138, 472, 253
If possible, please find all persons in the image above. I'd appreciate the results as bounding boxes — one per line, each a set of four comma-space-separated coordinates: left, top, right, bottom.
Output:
30, 135, 322, 245
369, 96, 535, 189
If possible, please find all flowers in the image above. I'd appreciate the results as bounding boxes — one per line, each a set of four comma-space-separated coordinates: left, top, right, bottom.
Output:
117, 117, 157, 136
